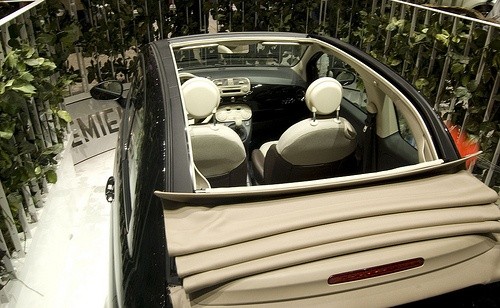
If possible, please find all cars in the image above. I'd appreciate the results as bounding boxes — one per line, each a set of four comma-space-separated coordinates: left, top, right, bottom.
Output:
88, 29, 499, 308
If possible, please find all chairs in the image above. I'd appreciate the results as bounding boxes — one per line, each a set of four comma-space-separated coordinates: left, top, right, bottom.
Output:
181, 77, 247, 188
251, 77, 359, 186
